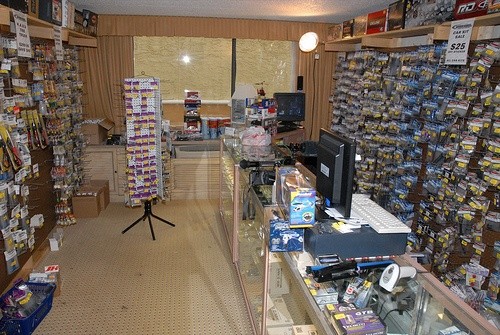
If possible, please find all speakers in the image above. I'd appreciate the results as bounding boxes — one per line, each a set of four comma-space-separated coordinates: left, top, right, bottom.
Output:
297, 76, 303, 93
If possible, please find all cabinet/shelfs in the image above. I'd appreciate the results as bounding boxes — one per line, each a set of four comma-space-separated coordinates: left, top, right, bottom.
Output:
0, 0, 99, 300
232, 164, 317, 335
171, 125, 306, 201
323, 13, 499, 334
83, 132, 171, 203
218, 132, 293, 264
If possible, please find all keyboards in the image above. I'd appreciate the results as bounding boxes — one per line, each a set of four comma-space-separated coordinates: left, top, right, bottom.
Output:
351, 194, 412, 234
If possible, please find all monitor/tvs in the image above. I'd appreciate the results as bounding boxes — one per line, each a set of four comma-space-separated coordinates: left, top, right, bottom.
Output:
316, 127, 357, 219
274, 93, 305, 122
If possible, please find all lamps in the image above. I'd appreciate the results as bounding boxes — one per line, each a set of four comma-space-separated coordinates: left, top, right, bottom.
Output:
298, 31, 320, 60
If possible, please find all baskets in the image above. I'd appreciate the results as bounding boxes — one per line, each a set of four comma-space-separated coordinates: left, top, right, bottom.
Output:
0, 282, 56, 335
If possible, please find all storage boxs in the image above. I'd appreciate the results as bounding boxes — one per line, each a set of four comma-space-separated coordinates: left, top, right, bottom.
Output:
81, 115, 116, 146
71, 189, 99, 218
80, 179, 110, 212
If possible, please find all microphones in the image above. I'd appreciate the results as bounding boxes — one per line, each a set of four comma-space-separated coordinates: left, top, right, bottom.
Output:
240, 159, 278, 169
